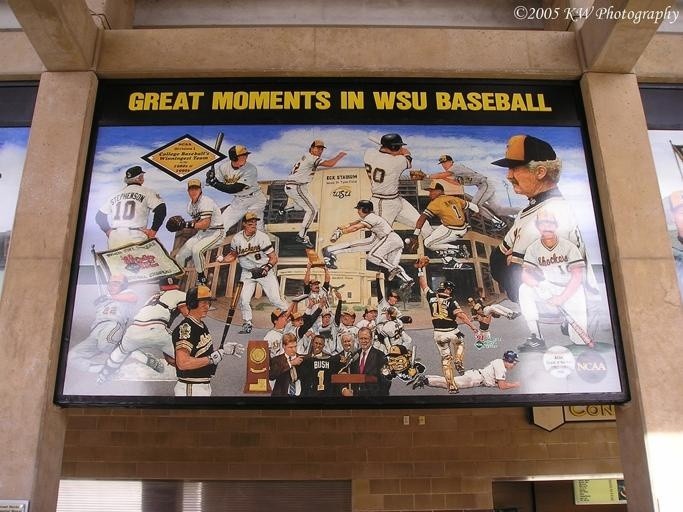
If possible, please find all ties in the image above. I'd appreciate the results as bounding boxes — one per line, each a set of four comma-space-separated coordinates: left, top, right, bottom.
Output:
359, 351, 366, 375
288, 356, 296, 397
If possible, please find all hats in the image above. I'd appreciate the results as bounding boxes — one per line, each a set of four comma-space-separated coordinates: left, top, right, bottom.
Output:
187, 178, 200, 190
271, 279, 398, 322
107, 273, 129, 285
310, 139, 327, 148
125, 165, 146, 179
424, 181, 443, 190
384, 345, 408, 358
228, 144, 252, 161
437, 154, 453, 165
537, 210, 555, 223
241, 212, 260, 222
490, 134, 556, 167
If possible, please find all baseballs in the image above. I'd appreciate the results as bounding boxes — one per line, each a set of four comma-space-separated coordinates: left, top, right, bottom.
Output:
404, 238, 411, 245
217, 256, 224, 262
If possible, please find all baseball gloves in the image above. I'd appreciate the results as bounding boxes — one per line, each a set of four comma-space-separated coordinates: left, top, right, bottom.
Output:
413, 254, 430, 268
166, 216, 185, 232
408, 169, 426, 180
248, 267, 269, 278
403, 234, 419, 253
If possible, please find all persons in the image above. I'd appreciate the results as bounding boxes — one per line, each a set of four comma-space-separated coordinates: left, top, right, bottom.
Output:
67, 134, 600, 397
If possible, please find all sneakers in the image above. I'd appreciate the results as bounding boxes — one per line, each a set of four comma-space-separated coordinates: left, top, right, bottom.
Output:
158, 275, 181, 291
387, 266, 401, 282
456, 244, 471, 259
412, 374, 427, 390
399, 277, 415, 290
195, 277, 212, 288
489, 220, 508, 233
448, 383, 460, 394
320, 244, 338, 270
145, 352, 165, 374
441, 257, 471, 270
295, 232, 315, 249
517, 333, 548, 353
454, 358, 464, 376
238, 320, 253, 334
559, 314, 569, 336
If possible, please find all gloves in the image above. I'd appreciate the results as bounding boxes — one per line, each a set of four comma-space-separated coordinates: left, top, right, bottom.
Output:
207, 348, 226, 366
223, 342, 245, 359
93, 293, 113, 313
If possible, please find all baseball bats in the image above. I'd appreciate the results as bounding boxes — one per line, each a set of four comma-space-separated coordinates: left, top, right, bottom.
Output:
369, 136, 380, 144
210, 282, 244, 374
206, 132, 224, 185
557, 305, 596, 349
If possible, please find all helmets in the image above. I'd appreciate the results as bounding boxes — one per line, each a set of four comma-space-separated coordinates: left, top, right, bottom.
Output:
437, 281, 456, 291
380, 133, 407, 148
185, 284, 218, 309
503, 351, 519, 364
353, 199, 373, 213
